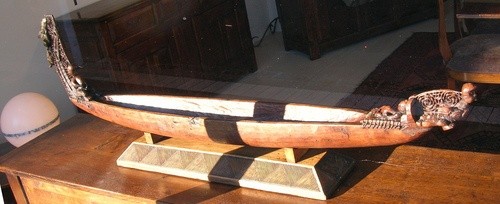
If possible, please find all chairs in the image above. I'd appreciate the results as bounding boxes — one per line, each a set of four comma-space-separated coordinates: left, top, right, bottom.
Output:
439, 0, 500, 91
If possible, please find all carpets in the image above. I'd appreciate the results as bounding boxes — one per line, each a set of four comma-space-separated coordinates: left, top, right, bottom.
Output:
335, 31, 500, 154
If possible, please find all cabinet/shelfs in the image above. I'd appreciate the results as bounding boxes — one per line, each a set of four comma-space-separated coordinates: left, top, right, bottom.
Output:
53, 0, 258, 114
274, 0, 440, 61
0, 111, 500, 204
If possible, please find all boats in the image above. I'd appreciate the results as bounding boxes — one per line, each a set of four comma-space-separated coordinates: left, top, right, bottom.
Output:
39, 12, 477, 150
